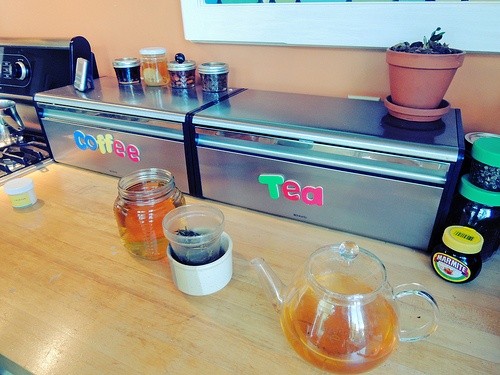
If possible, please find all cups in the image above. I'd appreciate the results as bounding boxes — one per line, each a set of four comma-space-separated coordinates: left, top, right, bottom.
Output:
161, 202, 224, 263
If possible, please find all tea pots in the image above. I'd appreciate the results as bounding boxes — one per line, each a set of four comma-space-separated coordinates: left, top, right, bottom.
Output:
248, 240, 441, 375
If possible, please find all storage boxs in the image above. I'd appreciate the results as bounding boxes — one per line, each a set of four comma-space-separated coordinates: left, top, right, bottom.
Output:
33, 76, 245, 196
189, 88, 467, 253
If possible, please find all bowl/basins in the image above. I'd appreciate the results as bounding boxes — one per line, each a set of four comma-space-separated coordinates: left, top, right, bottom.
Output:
166, 231, 234, 297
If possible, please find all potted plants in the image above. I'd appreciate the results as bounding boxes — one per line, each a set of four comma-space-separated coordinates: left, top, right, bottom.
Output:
386, 27, 465, 110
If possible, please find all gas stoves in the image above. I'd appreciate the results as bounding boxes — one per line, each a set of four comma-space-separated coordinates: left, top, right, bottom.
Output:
0, 135, 53, 181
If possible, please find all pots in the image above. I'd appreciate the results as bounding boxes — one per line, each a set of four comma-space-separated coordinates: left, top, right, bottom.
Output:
0, 99, 26, 143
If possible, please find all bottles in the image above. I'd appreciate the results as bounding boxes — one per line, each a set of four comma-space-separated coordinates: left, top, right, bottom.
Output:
470, 136, 500, 193
4, 178, 36, 208
452, 173, 500, 262
197, 61, 229, 92
430, 225, 484, 284
112, 167, 186, 261
139, 48, 171, 87
166, 60, 196, 89
112, 57, 141, 85
